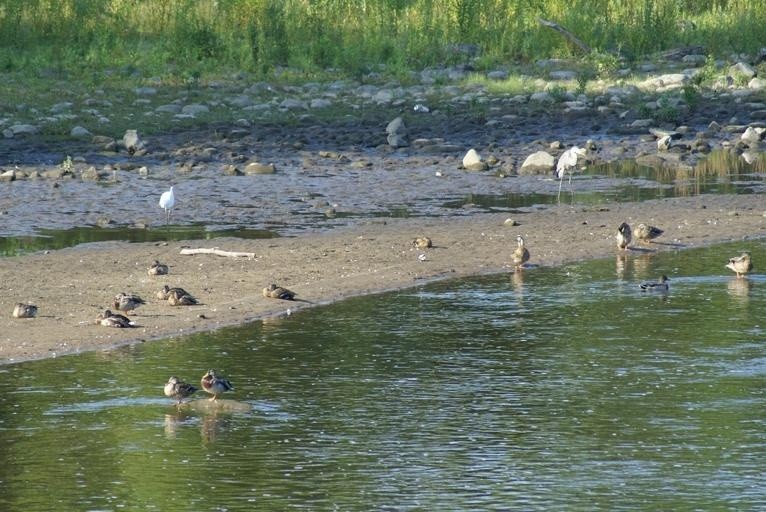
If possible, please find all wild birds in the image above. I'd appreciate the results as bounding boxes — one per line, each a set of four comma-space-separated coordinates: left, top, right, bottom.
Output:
633, 223, 664, 244
201, 368, 237, 402
263, 283, 298, 300
639, 274, 670, 292
412, 236, 432, 248
159, 186, 174, 225
157, 285, 187, 300
115, 293, 147, 315
148, 260, 168, 275
101, 310, 136, 328
616, 223, 632, 250
724, 250, 753, 279
168, 291, 199, 306
550, 145, 585, 192
163, 376, 202, 404
13, 303, 37, 318
510, 235, 529, 269
